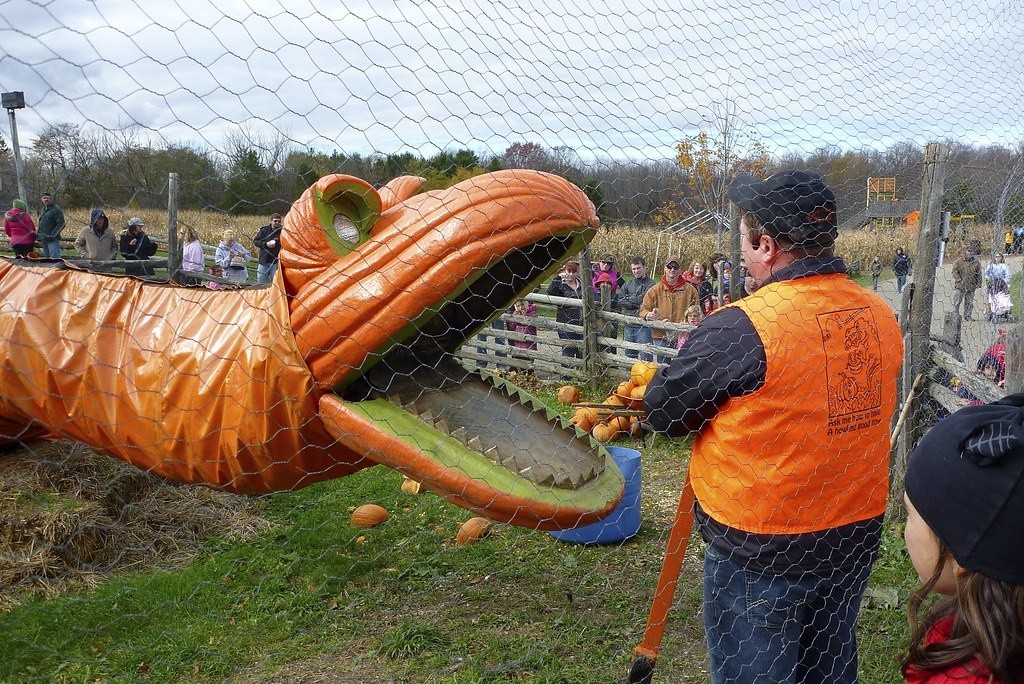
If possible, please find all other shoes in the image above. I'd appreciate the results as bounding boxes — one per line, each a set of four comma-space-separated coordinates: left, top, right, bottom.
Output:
964, 316, 976, 322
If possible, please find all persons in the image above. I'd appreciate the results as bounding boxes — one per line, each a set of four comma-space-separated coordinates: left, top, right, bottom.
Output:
507, 299, 537, 376
984, 252, 1011, 287
506, 284, 541, 374
617, 256, 658, 362
120, 218, 159, 277
954, 329, 1008, 408
35, 192, 66, 258
73, 208, 119, 274
205, 266, 232, 290
3, 199, 37, 256
894, 392, 1024, 684
475, 314, 506, 371
951, 247, 983, 322
1004, 222, 1024, 256
870, 255, 882, 292
673, 252, 760, 350
639, 164, 907, 684
639, 259, 700, 365
591, 251, 626, 370
215, 230, 251, 283
892, 247, 912, 292
253, 213, 284, 285
546, 262, 584, 381
177, 224, 203, 285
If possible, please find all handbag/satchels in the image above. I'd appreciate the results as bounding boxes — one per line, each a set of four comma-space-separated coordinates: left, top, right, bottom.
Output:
230, 251, 246, 270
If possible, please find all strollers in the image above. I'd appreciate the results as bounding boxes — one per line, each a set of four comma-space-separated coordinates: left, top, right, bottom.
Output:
983, 277, 1015, 325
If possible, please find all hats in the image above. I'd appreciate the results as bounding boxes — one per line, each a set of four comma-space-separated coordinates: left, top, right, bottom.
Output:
904, 392, 1024, 586
594, 273, 612, 288
13, 199, 26, 213
129, 218, 145, 226
728, 169, 840, 249
665, 256, 681, 267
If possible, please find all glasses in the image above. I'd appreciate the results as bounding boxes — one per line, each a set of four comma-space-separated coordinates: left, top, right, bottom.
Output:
666, 263, 681, 270
180, 231, 185, 234
272, 220, 281, 223
601, 260, 614, 265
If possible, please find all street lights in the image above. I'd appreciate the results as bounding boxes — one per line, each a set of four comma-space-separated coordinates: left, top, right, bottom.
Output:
0, 91, 30, 205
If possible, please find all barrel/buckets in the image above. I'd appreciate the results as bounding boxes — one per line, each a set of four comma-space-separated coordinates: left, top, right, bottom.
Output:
548, 446, 642, 545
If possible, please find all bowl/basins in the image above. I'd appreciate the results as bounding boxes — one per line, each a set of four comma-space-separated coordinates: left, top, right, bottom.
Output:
612, 390, 645, 410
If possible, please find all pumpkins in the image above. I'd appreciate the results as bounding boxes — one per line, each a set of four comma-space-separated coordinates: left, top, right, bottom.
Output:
558, 362, 660, 444
350, 504, 390, 527
457, 518, 491, 545
401, 479, 426, 494
